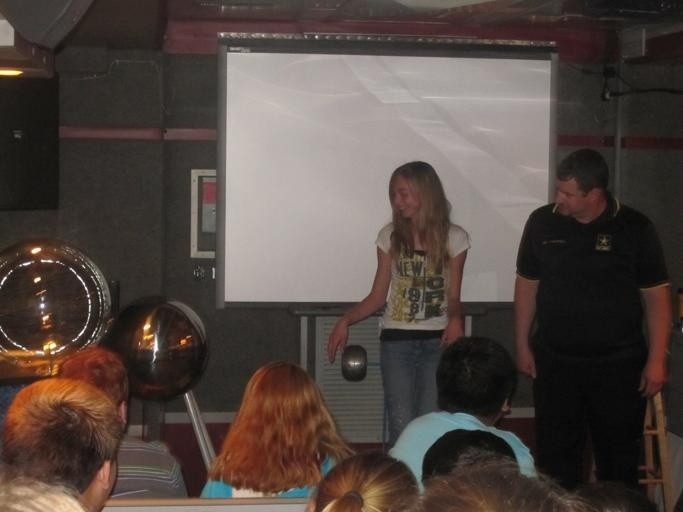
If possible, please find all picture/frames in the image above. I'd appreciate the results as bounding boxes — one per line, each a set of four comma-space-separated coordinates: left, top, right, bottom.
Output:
186, 168, 216, 263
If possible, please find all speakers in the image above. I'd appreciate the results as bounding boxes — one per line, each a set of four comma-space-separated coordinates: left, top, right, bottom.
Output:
0, 61, 60, 212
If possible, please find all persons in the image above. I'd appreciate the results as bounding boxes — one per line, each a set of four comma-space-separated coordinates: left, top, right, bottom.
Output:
387, 333, 543, 494
194, 357, 356, 502
304, 453, 419, 512
55, 346, 190, 499
418, 430, 522, 489
1, 375, 127, 512
323, 160, 475, 455
565, 482, 657, 512
393, 454, 584, 512
512, 147, 672, 492
